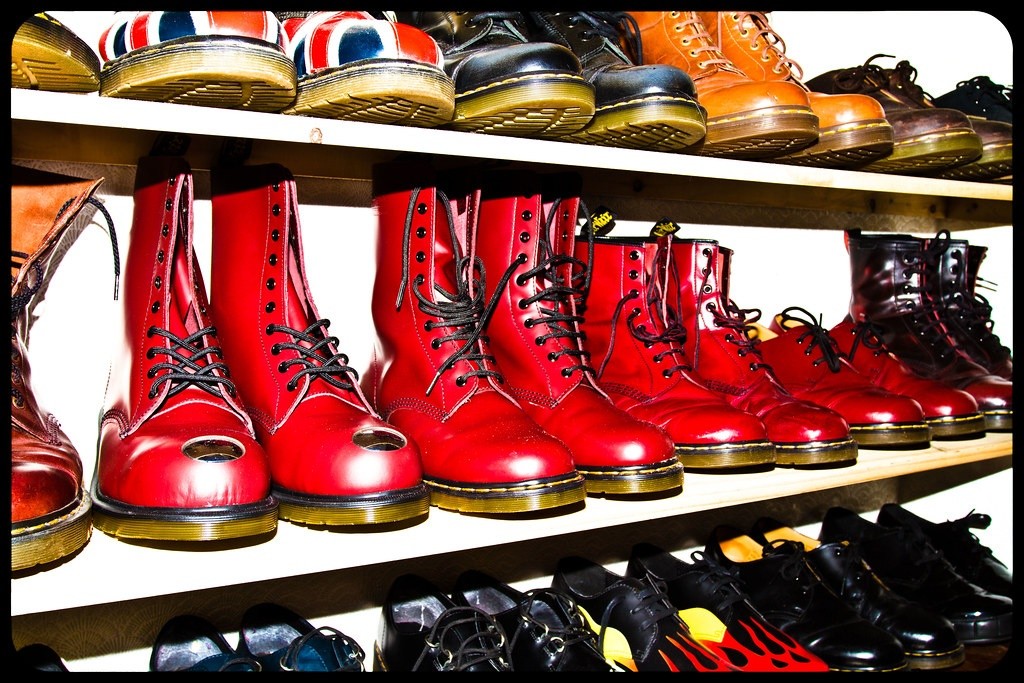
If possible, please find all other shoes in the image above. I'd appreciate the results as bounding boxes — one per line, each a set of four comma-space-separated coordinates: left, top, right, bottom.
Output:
19, 645, 67, 673
12, 10, 101, 94
98, 10, 456, 128
152, 603, 368, 673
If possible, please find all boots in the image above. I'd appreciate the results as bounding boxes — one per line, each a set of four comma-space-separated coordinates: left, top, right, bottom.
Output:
10, 164, 120, 573
622, 10, 896, 168
842, 228, 1013, 431
358, 183, 685, 517
90, 154, 427, 545
572, 234, 858, 467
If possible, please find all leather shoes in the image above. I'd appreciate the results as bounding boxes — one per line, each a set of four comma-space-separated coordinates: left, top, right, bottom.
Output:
818, 503, 1013, 646
550, 544, 830, 673
402, 9, 706, 150
708, 519, 967, 673
804, 51, 1012, 181
373, 572, 614, 673
747, 304, 986, 448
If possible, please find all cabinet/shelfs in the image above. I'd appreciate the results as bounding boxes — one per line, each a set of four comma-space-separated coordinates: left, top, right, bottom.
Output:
1, 80, 1015, 616
